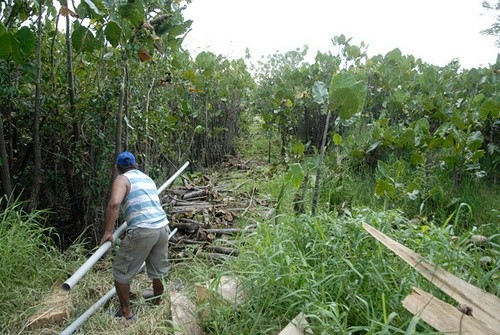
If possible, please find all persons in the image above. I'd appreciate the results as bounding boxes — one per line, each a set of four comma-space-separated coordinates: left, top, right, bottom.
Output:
100, 150, 170, 319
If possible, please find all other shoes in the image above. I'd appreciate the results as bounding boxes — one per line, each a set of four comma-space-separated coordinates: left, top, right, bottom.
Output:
113, 292, 157, 319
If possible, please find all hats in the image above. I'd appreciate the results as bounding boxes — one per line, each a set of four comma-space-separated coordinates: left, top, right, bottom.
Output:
115, 151, 136, 165
